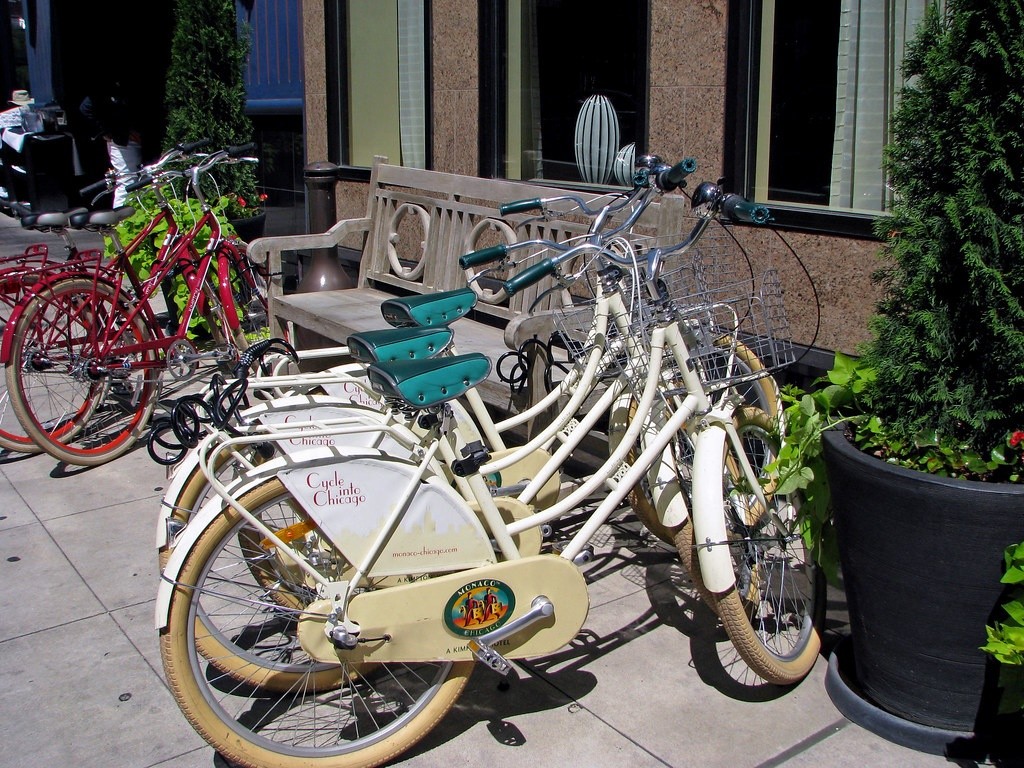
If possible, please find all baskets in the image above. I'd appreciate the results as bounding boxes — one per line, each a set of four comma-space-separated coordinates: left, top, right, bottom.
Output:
580, 220, 737, 305
553, 259, 719, 378
605, 268, 794, 403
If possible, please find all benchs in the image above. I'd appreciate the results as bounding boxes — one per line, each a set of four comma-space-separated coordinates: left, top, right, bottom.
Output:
248, 154, 685, 466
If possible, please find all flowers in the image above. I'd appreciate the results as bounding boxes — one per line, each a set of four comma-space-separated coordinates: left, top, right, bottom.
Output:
777, 0, 1024, 665
103, 0, 269, 341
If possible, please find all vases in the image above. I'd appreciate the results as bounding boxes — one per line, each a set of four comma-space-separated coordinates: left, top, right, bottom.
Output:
818, 416, 1024, 729
159, 212, 265, 339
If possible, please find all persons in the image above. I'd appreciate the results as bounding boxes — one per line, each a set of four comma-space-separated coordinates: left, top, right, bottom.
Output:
0, 90, 33, 115
81, 71, 143, 208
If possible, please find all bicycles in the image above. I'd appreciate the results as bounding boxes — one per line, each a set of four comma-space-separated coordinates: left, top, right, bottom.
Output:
153, 151, 830, 768
0, 146, 293, 473
